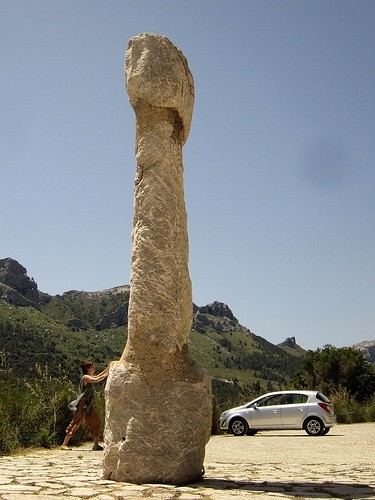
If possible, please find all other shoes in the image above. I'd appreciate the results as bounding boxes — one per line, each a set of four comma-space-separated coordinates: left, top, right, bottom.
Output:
61, 445, 72, 450
93, 445, 103, 450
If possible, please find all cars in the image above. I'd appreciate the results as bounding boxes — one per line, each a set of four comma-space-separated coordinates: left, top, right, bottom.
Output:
219, 390, 335, 436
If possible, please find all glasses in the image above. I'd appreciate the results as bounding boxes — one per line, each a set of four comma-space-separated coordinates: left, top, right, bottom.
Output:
89, 366, 95, 369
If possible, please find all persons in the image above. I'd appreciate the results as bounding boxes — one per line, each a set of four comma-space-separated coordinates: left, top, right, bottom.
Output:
60, 357, 112, 450
